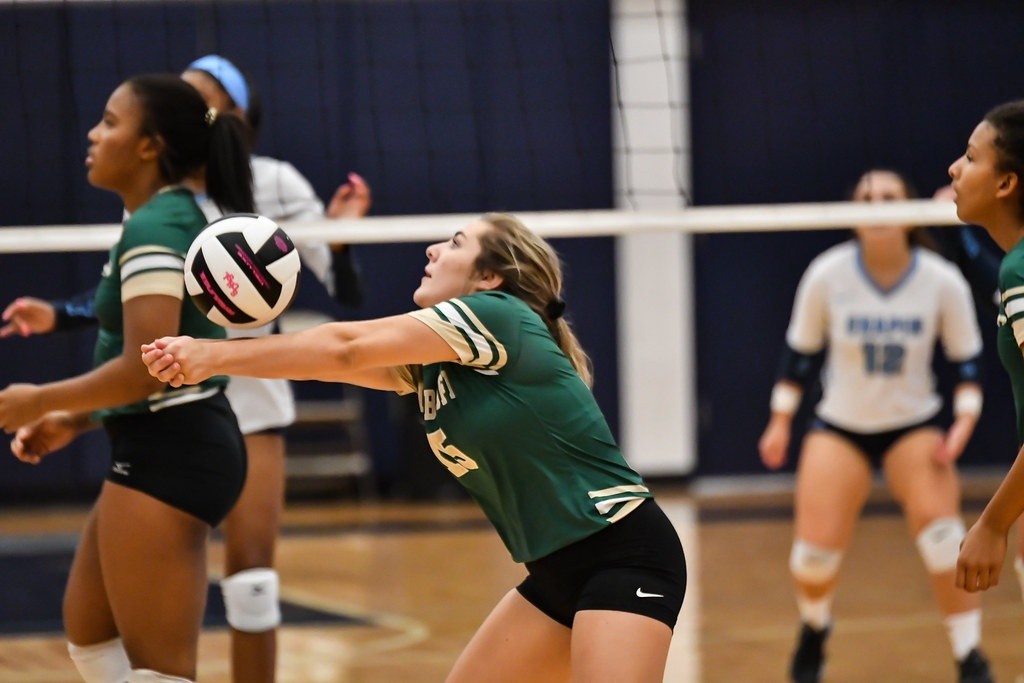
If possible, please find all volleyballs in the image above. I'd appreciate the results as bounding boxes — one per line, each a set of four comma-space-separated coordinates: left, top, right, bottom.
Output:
183, 209, 303, 331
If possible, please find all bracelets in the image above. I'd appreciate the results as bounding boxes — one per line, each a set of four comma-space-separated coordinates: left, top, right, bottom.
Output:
770, 385, 799, 416
954, 390, 983, 417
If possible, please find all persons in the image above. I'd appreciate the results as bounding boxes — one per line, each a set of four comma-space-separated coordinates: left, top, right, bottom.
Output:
759, 170, 993, 683
0, 53, 370, 683
948, 102, 1024, 593
142, 213, 686, 683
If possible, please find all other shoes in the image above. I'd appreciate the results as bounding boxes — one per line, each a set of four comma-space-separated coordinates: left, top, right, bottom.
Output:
959, 650, 991, 683
792, 625, 826, 683
